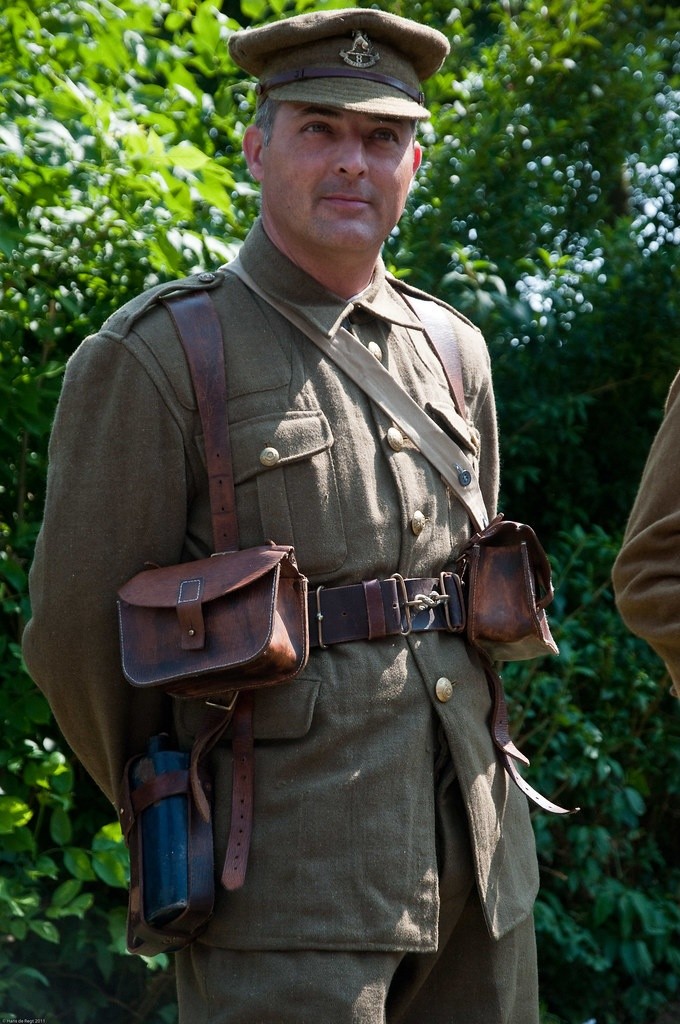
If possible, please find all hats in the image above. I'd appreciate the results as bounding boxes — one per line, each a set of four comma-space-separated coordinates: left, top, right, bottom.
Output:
229, 8, 451, 118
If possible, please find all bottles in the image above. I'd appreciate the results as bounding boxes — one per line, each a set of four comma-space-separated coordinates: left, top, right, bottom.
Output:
124, 732, 217, 958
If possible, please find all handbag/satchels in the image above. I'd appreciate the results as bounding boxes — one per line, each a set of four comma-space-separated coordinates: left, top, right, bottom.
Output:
462, 513, 559, 664
117, 536, 310, 701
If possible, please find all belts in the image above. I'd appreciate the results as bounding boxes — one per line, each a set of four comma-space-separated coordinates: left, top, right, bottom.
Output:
311, 574, 466, 650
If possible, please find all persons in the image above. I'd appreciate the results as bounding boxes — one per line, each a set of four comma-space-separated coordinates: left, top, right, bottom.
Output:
610, 357, 680, 694
20, 9, 556, 1024
